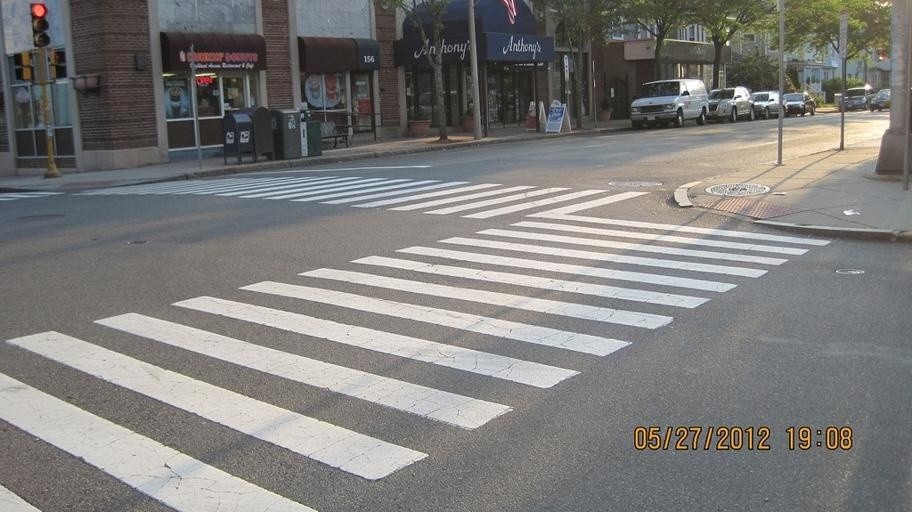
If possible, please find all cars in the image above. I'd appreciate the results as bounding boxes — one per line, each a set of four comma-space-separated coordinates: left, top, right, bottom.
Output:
407, 92, 431, 120
839, 87, 890, 112
709, 86, 816, 123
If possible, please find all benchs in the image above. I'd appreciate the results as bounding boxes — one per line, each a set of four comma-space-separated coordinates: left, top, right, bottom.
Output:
321, 119, 349, 149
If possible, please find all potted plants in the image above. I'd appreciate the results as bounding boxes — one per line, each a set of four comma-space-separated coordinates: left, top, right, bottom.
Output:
598, 98, 615, 121
408, 110, 432, 138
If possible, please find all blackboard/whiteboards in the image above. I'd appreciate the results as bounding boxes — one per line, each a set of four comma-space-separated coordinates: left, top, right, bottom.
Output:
546, 104, 566, 132
525, 101, 543, 130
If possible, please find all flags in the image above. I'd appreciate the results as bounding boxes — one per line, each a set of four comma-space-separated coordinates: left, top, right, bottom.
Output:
499, 0, 521, 25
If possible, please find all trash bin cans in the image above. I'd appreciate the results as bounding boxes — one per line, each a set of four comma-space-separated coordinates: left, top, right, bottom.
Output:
222, 105, 322, 165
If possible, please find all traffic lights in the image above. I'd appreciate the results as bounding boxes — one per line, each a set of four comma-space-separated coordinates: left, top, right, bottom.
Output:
30, 2, 50, 47
13, 51, 32, 82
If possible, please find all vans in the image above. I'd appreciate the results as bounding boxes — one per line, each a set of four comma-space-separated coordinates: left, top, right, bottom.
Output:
631, 79, 710, 130
